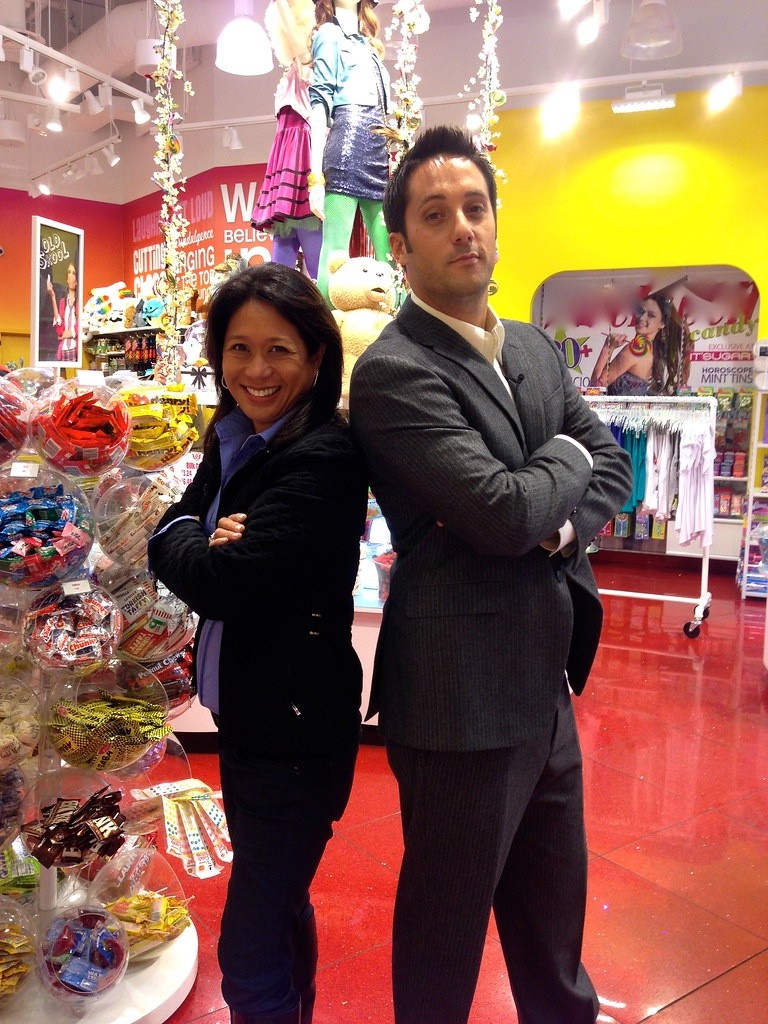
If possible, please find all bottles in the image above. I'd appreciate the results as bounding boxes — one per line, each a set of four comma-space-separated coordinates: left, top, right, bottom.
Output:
96, 353, 109, 377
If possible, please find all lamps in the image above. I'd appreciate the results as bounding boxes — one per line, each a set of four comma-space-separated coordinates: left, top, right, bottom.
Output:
98, 83, 112, 106
131, 98, 150, 124
26, 53, 46, 85
620, 0, 684, 63
102, 145, 120, 168
66, 68, 80, 92
611, 83, 676, 115
224, 127, 244, 150
0, 100, 27, 148
89, 157, 104, 175
84, 90, 105, 115
19, 45, 34, 73
215, 0, 274, 76
134, 0, 177, 78
39, 172, 57, 196
46, 110, 62, 132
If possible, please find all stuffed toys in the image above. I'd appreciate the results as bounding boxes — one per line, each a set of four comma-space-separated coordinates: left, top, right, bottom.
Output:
327, 249, 396, 398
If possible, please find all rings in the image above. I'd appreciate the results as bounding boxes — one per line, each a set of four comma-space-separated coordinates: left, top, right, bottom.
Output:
208, 532, 215, 543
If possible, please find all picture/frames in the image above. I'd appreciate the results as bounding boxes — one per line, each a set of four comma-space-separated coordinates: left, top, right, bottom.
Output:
27, 216, 84, 367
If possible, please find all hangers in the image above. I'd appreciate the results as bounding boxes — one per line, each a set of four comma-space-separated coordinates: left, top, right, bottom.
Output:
590, 394, 711, 429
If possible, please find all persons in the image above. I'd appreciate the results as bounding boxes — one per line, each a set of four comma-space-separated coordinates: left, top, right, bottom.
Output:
590, 294, 693, 396
350, 127, 634, 1024
264, 0, 407, 310
47, 262, 79, 362
148, 263, 367, 1024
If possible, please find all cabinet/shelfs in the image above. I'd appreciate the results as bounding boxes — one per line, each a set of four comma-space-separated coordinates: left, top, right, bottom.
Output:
578, 383, 751, 561
740, 391, 767, 600
